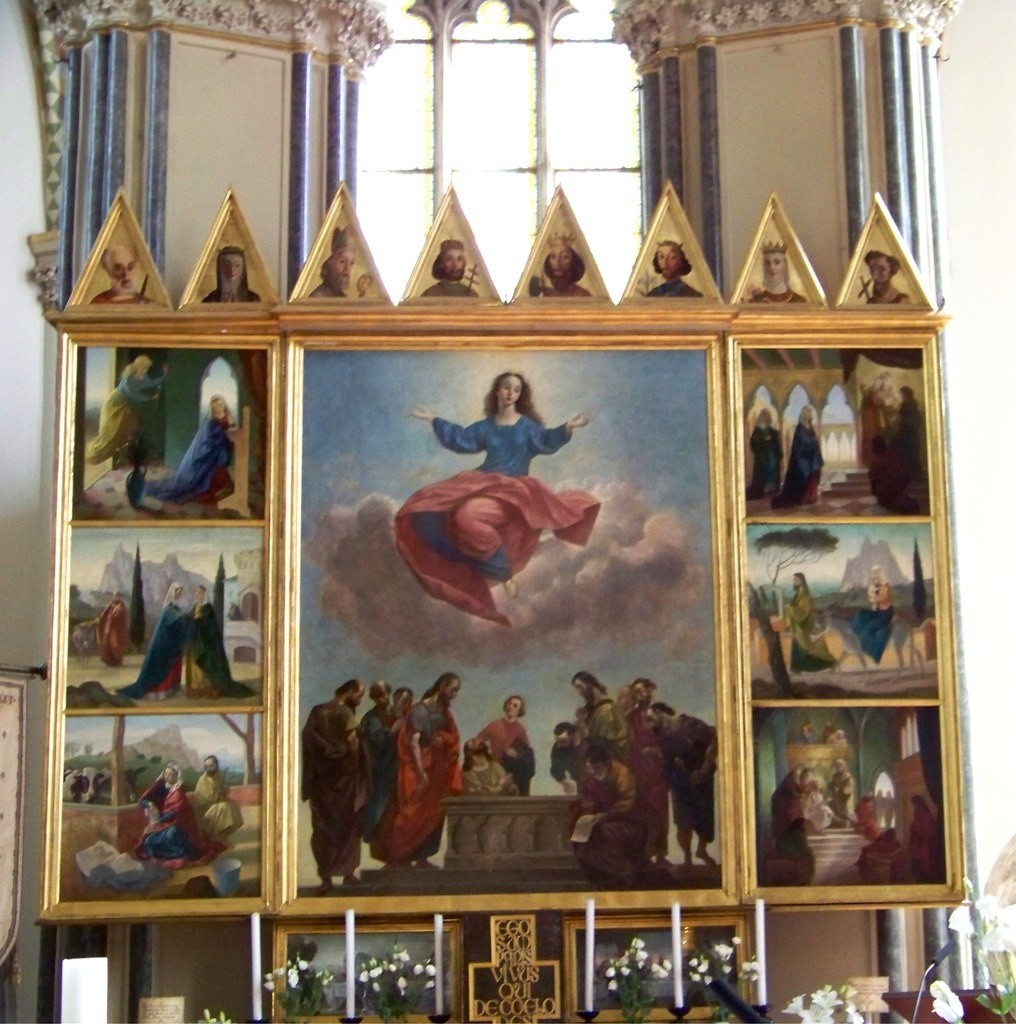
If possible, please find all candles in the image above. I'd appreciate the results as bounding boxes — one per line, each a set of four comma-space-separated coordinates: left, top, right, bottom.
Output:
584, 896, 596, 1013
670, 899, 685, 1010
345, 907, 358, 1016
249, 912, 263, 1021
434, 912, 444, 1016
754, 896, 770, 1004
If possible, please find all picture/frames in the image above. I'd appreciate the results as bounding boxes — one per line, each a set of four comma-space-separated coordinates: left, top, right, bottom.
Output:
289, 336, 728, 913
56, 334, 282, 525
735, 523, 954, 703
731, 709, 963, 910
563, 911, 754, 1024
730, 328, 945, 516
47, 717, 280, 913
273, 922, 466, 1024
54, 531, 277, 709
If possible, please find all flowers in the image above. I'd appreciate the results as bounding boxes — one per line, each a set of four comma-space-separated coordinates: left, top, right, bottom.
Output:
265, 955, 329, 1008
930, 877, 1016, 1024
355, 948, 432, 1015
779, 984, 865, 1023
602, 936, 667, 1011
685, 939, 747, 1019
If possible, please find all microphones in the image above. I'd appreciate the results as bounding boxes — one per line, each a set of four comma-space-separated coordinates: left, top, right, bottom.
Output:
910, 941, 957, 1024
710, 979, 768, 1024
